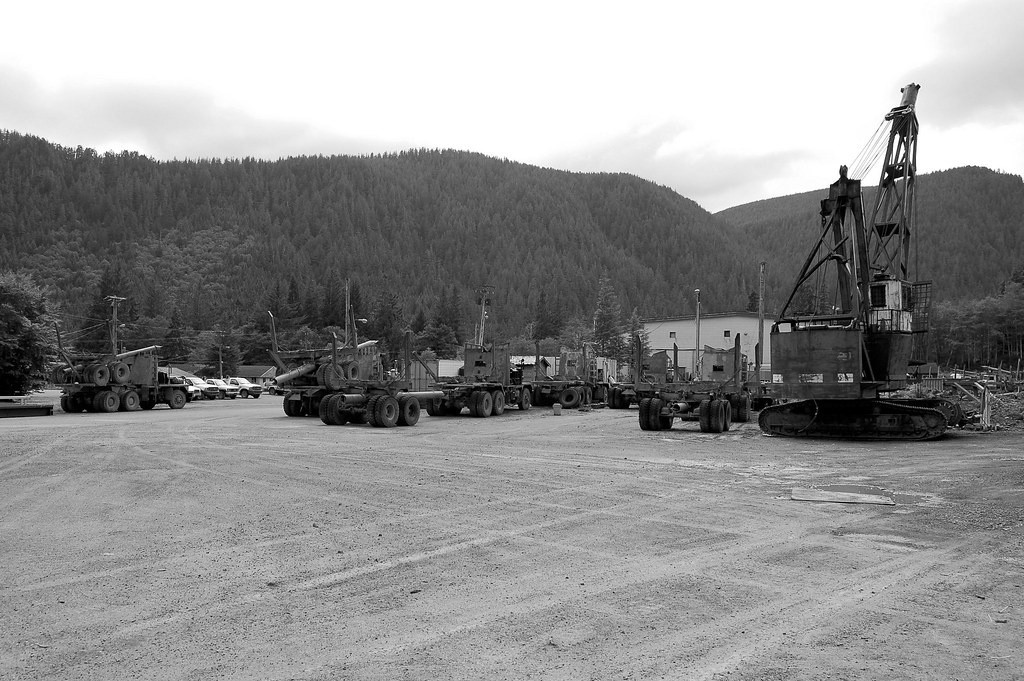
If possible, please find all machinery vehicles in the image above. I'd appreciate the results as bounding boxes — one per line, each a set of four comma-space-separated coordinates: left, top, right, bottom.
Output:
758, 78, 965, 440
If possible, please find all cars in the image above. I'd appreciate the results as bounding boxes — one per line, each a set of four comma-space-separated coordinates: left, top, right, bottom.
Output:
177, 378, 202, 401
206, 379, 240, 401
263, 380, 284, 395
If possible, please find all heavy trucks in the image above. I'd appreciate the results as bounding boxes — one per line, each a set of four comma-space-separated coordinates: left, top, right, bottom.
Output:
631, 333, 754, 433
60, 344, 189, 413
264, 309, 628, 428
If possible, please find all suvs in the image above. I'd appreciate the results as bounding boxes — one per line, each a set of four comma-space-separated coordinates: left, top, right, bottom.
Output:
184, 376, 219, 400
226, 378, 263, 398
